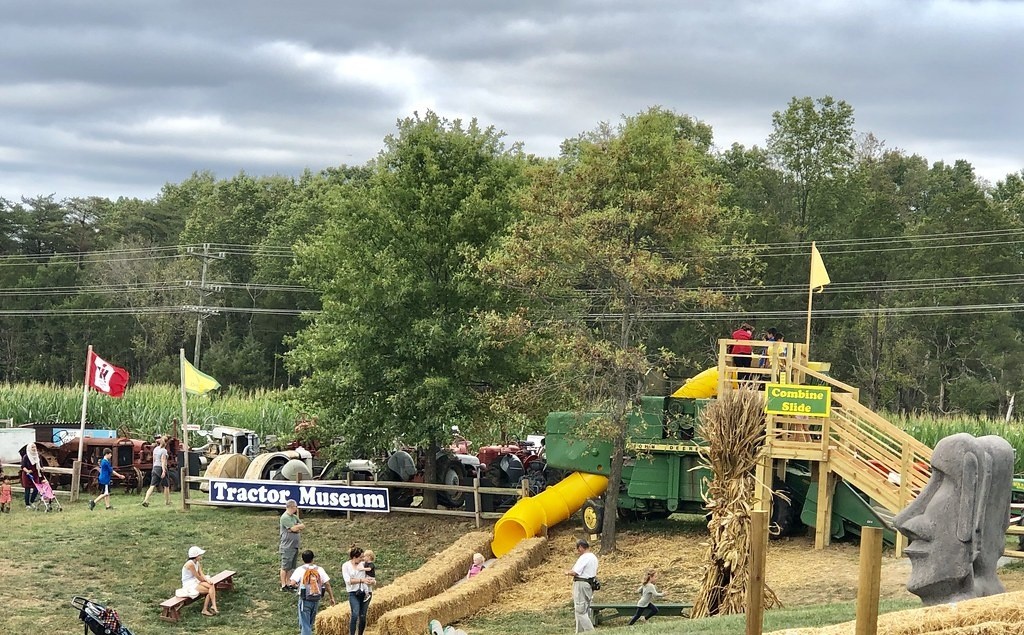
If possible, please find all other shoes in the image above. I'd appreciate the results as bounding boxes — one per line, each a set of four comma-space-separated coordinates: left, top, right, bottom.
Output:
203, 611, 213, 616
211, 607, 220, 613
363, 594, 370, 603
282, 586, 290, 591
142, 502, 149, 506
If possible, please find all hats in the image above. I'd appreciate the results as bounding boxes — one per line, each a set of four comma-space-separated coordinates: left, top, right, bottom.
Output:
189, 546, 206, 558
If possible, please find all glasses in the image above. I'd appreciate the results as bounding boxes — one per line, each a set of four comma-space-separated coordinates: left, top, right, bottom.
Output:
292, 505, 298, 508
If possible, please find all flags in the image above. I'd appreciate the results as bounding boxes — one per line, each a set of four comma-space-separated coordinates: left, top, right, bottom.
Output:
85, 350, 129, 397
810, 244, 830, 289
181, 356, 221, 395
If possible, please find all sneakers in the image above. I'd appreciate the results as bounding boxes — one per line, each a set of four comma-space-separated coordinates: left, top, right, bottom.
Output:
90, 500, 96, 511
107, 506, 116, 511
31, 504, 39, 510
26, 505, 33, 510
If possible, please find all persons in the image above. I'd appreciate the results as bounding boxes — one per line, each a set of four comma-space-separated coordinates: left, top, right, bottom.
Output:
728, 323, 787, 390
0, 475, 12, 515
357, 549, 375, 602
280, 499, 306, 592
627, 569, 668, 626
342, 546, 377, 635
563, 538, 598, 631
182, 546, 219, 615
22, 441, 45, 510
142, 436, 173, 507
290, 550, 335, 634
467, 553, 486, 578
89, 448, 125, 510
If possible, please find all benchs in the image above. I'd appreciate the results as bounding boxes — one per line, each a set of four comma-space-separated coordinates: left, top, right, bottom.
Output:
160, 570, 237, 622
590, 603, 694, 626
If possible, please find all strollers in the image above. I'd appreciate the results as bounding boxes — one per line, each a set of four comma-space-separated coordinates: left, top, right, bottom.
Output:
26, 467, 63, 514
71, 596, 132, 635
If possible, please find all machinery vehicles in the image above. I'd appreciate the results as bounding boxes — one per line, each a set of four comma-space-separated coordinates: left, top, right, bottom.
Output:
18, 413, 342, 496
540, 337, 937, 550
377, 423, 480, 510
477, 433, 571, 510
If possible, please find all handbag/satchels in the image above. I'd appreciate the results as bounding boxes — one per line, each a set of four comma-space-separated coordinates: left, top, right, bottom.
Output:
589, 577, 600, 590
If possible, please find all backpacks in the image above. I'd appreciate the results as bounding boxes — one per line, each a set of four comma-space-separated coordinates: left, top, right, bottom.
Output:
301, 566, 323, 601
